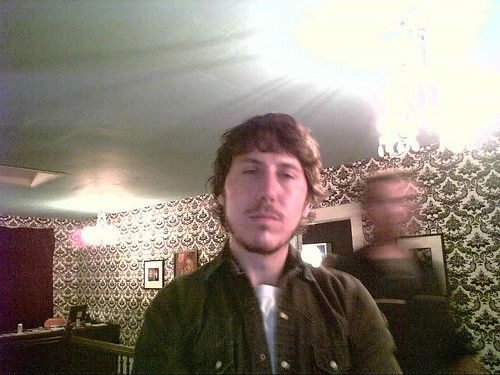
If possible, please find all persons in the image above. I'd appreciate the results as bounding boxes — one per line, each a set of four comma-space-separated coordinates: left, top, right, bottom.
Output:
340, 168, 473, 375
131, 113, 402, 375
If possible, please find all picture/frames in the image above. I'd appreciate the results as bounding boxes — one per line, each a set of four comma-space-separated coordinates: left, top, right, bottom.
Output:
143, 259, 165, 290
173, 248, 198, 278
394, 233, 450, 298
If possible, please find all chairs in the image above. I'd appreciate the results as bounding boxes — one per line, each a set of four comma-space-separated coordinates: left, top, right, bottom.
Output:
9, 320, 29, 329
31, 315, 50, 329
44, 317, 67, 328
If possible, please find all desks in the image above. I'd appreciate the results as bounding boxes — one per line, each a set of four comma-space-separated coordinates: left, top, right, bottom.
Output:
0, 321, 120, 375
